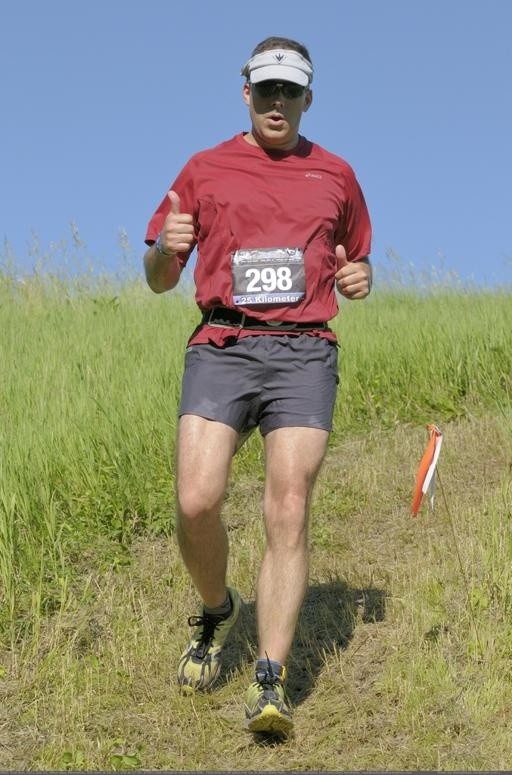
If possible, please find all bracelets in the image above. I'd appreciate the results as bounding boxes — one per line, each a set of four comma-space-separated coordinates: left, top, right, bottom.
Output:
154, 235, 179, 258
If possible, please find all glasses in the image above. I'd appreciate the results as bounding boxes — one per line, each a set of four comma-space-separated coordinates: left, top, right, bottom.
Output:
256, 81, 303, 98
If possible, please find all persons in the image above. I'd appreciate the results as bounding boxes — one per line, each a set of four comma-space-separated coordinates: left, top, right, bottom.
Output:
142, 33, 376, 740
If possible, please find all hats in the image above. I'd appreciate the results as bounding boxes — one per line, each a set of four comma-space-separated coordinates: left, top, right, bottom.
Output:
240, 49, 315, 89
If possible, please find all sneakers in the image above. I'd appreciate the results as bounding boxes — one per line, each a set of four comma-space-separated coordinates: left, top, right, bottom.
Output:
242, 652, 293, 733
178, 588, 242, 697
